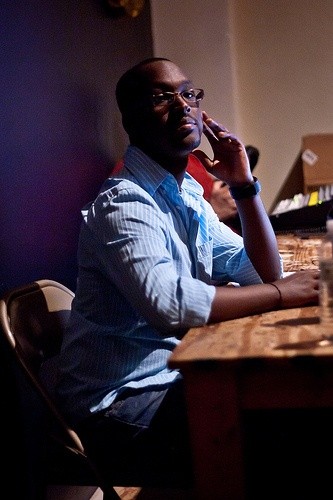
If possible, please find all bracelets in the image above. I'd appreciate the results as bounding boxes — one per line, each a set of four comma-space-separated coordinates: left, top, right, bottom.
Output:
269, 282, 282, 306
228, 176, 261, 200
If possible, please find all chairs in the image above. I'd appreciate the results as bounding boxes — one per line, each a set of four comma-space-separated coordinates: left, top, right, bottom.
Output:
0, 280, 189, 500
302, 132, 333, 195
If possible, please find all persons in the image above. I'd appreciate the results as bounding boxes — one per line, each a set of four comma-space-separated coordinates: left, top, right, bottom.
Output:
53, 58, 333, 500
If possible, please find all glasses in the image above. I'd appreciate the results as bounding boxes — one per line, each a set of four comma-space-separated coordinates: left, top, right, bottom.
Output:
152, 88, 204, 106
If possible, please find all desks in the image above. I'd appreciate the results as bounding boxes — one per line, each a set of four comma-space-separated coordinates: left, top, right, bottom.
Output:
167, 233, 333, 500
